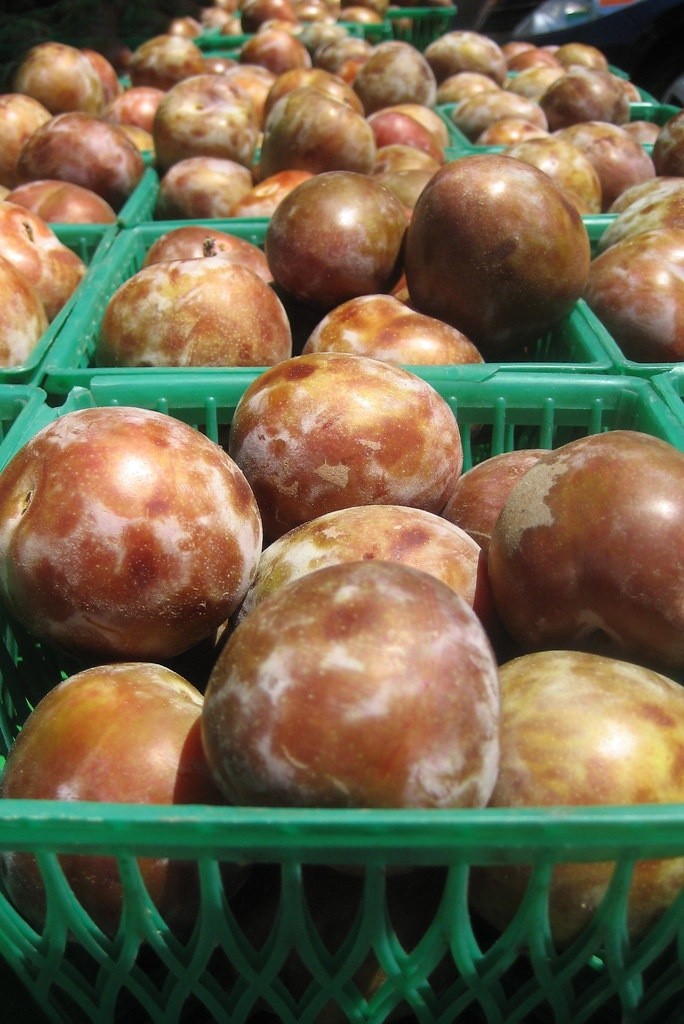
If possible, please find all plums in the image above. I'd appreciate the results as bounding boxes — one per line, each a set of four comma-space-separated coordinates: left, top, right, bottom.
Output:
0, 0, 684, 948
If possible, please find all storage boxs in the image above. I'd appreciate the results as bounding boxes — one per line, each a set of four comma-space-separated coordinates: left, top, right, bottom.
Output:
0, 0, 684, 1024
